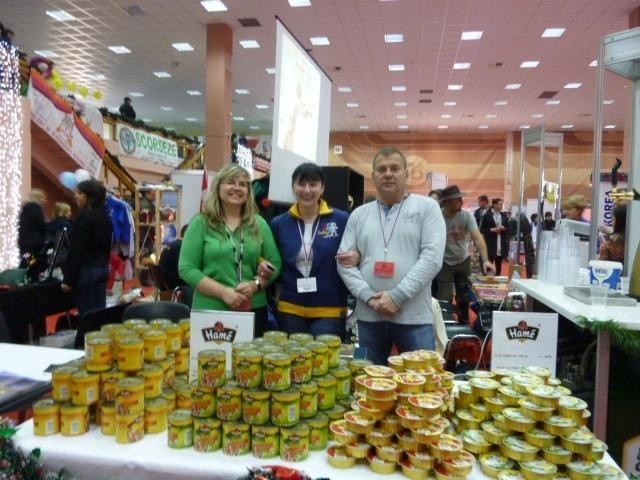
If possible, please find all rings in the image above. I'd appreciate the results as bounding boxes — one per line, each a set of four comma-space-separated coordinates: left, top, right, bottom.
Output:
260, 271, 264, 276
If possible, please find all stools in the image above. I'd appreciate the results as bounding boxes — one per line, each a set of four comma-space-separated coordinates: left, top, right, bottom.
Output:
433, 273, 510, 372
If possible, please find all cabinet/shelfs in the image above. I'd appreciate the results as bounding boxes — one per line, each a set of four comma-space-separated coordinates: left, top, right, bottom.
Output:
133, 181, 182, 286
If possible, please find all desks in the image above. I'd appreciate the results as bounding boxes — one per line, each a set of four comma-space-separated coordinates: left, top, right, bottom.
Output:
508, 275, 639, 445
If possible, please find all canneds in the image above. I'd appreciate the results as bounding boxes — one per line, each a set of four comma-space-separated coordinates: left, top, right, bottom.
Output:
167, 330, 374, 463
327, 349, 475, 480
447, 366, 622, 479
33, 318, 191, 445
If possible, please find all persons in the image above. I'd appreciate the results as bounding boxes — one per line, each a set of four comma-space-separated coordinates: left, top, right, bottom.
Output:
257, 161, 362, 344
562, 192, 593, 243
176, 162, 283, 339
428, 183, 558, 327
17, 169, 190, 328
334, 146, 448, 369
599, 204, 627, 262
118, 96, 137, 121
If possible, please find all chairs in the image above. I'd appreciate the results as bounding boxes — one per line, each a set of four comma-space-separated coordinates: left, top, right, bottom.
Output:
71, 301, 190, 353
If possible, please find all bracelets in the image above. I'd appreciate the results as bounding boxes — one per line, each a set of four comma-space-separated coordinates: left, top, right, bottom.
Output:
254, 279, 261, 292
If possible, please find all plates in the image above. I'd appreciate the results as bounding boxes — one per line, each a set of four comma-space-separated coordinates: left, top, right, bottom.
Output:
163, 224, 177, 243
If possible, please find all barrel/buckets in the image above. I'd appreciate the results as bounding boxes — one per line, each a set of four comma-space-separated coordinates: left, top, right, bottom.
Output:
588, 259, 624, 291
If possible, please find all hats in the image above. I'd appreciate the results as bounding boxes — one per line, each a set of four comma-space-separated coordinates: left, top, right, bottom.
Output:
438, 185, 469, 205
559, 194, 594, 211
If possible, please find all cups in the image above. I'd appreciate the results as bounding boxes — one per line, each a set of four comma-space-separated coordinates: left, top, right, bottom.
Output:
588, 285, 609, 317
536, 220, 582, 287
